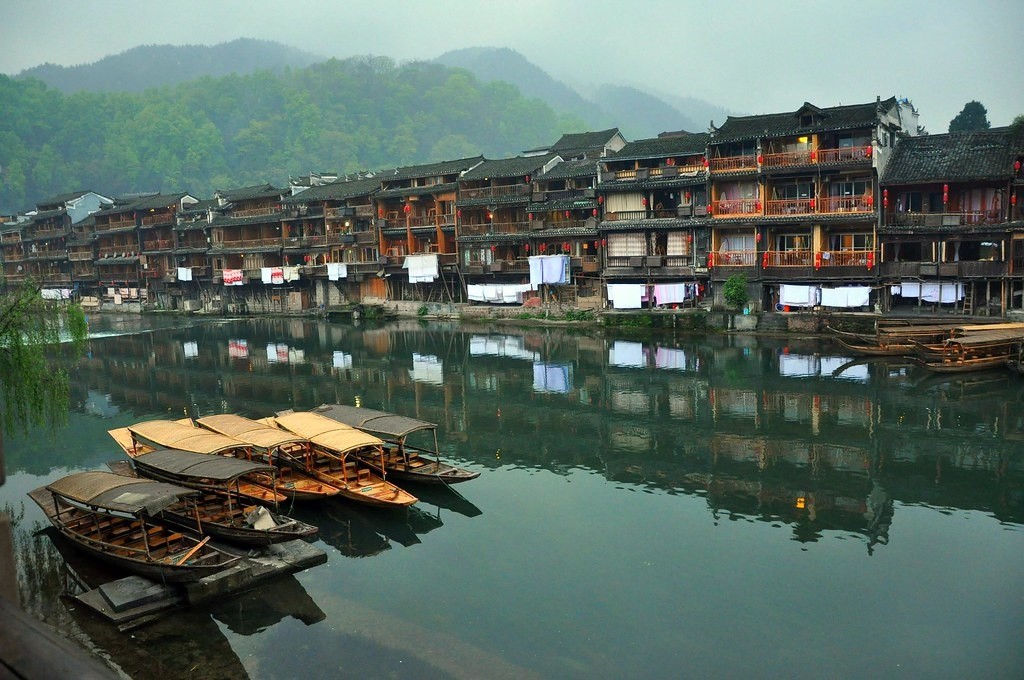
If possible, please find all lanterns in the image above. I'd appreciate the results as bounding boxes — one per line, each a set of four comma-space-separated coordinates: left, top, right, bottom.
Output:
1011, 161, 1021, 207
4, 145, 948, 312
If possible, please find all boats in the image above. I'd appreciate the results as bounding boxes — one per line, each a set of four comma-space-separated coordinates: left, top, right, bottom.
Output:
27, 470, 243, 583
825, 317, 1024, 373
44, 459, 322, 549
106, 404, 482, 509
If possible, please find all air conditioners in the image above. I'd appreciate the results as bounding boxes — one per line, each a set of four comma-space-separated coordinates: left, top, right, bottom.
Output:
178, 256, 186, 262
179, 232, 184, 236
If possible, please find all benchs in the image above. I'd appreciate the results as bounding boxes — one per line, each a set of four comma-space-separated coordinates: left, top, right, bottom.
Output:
65, 443, 426, 561
